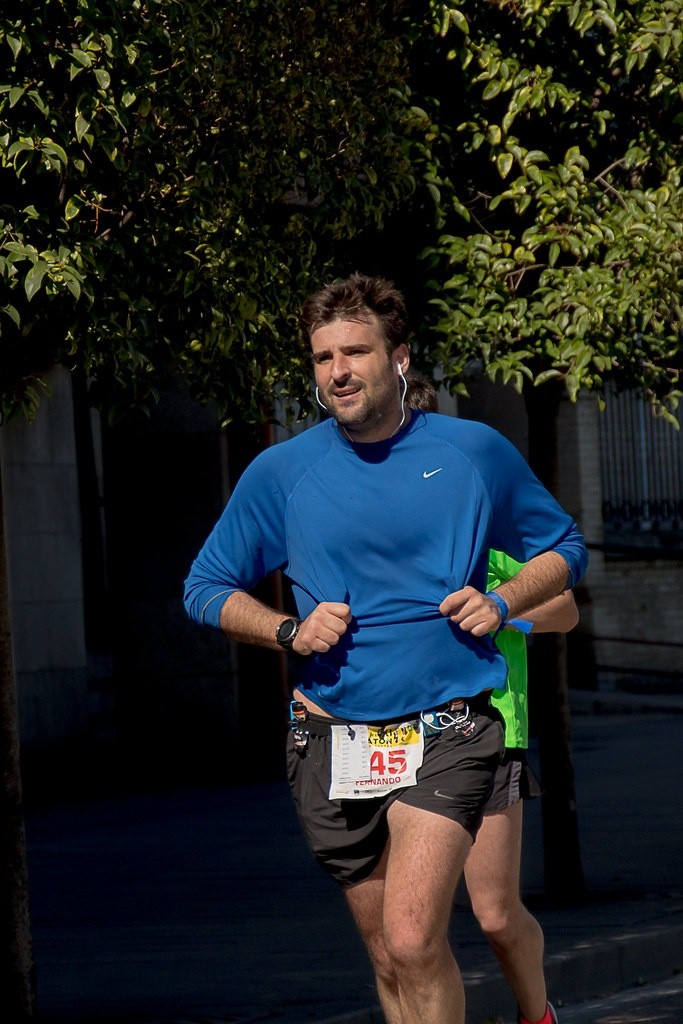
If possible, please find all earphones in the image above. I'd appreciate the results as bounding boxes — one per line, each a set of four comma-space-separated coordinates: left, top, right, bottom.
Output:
397, 362, 403, 376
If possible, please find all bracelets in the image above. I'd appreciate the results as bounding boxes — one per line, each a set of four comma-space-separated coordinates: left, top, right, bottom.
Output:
486, 591, 535, 640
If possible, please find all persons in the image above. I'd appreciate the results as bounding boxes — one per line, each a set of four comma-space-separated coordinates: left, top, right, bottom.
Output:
182, 271, 591, 1024
463, 548, 580, 1024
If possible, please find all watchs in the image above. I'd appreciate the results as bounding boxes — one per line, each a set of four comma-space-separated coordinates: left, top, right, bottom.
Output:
275, 618, 303, 652
